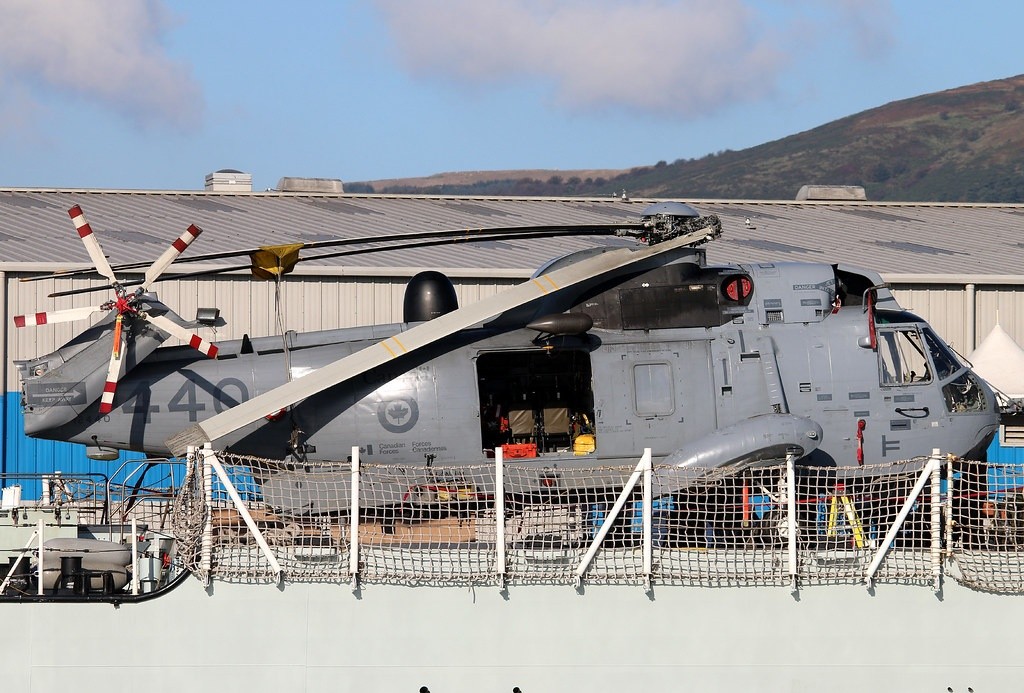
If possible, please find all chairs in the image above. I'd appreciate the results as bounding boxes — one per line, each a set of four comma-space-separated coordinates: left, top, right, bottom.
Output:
540, 407, 572, 453
508, 409, 539, 451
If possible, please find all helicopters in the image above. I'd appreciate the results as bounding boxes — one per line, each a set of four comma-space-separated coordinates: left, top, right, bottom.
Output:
13, 202, 1004, 540
